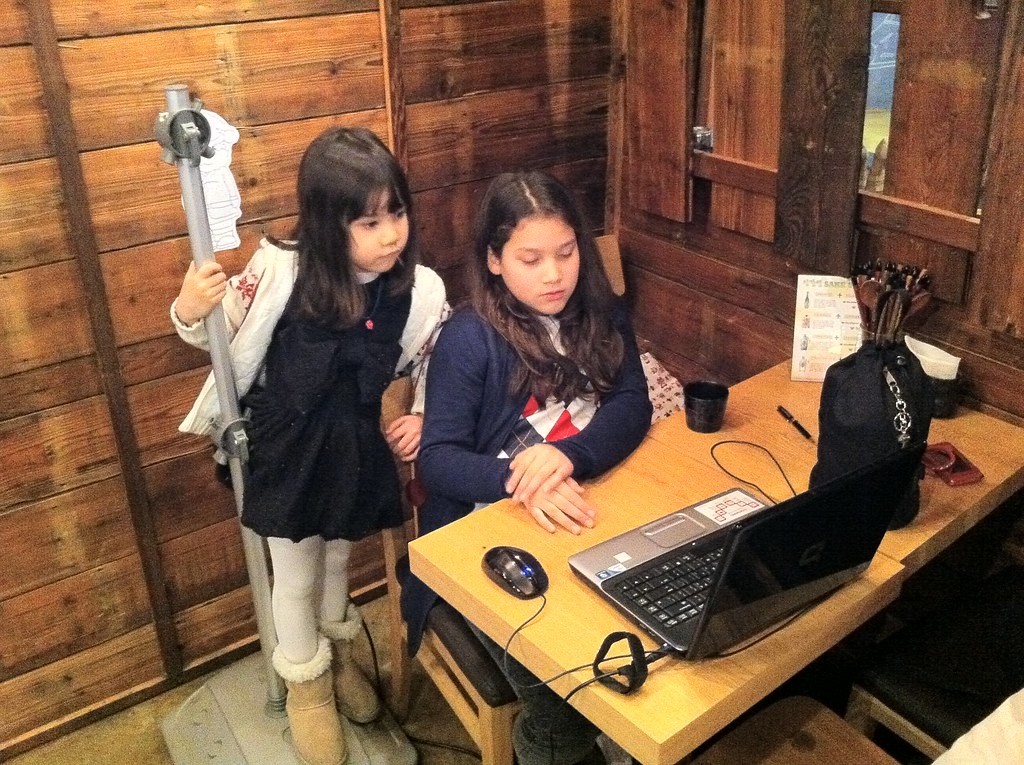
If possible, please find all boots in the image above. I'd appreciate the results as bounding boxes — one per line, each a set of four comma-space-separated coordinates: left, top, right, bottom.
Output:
272, 632, 348, 765
316, 602, 381, 725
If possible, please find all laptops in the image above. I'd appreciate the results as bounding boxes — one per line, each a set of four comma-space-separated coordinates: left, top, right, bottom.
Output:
567, 438, 930, 661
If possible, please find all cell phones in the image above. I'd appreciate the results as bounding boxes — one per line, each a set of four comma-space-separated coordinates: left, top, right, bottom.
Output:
924, 442, 982, 486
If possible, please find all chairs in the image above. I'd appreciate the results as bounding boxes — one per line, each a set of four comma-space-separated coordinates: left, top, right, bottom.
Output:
687, 684, 1024, 765
381, 525, 523, 765
595, 235, 627, 298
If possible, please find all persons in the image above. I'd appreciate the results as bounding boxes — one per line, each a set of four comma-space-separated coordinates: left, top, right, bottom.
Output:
415, 170, 653, 765
170, 125, 450, 765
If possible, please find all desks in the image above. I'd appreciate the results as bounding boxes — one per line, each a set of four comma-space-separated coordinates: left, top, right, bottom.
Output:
409, 432, 906, 765
657, 358, 1023, 590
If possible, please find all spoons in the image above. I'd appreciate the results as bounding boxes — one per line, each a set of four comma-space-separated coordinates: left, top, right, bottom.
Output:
897, 293, 931, 334
860, 280, 886, 332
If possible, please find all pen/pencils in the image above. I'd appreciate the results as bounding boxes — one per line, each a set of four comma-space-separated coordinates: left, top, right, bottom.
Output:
849, 257, 932, 333
776, 405, 815, 444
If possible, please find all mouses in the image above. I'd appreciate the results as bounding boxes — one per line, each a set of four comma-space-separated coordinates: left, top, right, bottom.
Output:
481, 546, 549, 600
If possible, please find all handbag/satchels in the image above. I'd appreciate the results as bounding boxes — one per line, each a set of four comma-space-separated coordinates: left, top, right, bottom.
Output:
812, 337, 934, 526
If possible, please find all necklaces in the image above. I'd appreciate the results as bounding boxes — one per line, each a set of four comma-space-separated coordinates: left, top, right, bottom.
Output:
362, 278, 382, 330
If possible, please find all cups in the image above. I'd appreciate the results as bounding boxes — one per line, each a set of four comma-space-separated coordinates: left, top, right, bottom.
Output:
683, 380, 729, 433
